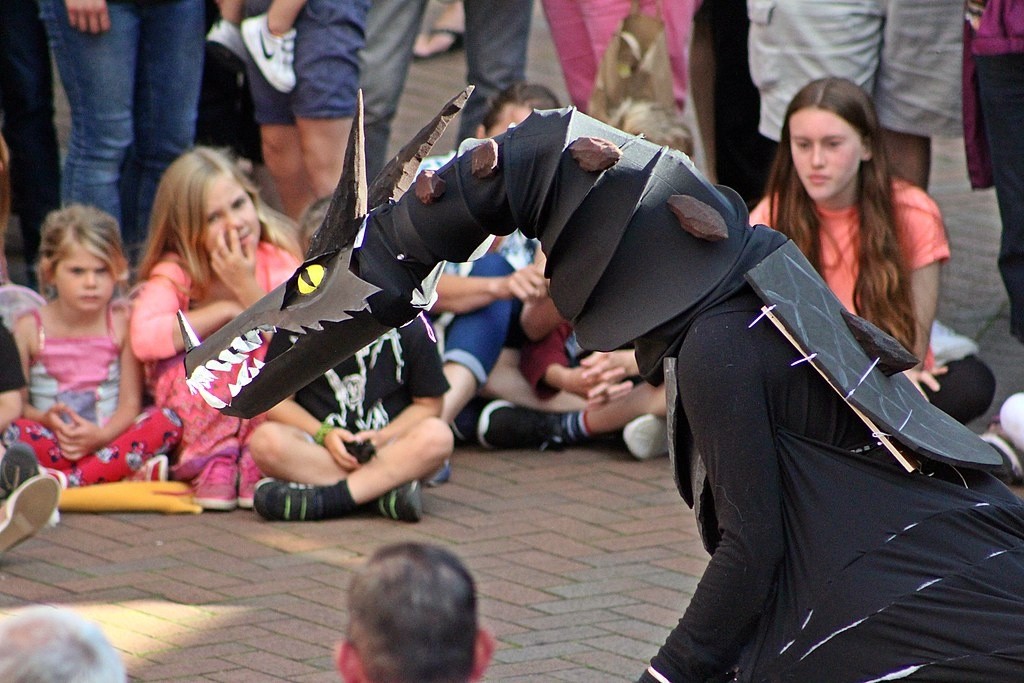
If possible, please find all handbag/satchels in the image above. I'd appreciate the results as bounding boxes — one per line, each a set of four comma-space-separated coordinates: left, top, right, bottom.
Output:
587, 0, 695, 166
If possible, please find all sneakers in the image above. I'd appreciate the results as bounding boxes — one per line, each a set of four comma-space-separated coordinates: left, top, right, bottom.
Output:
372, 477, 423, 522
241, 14, 296, 92
236, 454, 267, 509
251, 476, 325, 523
189, 455, 239, 510
206, 20, 248, 64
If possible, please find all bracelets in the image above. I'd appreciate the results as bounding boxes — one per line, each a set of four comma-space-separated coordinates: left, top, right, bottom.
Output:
314, 421, 334, 446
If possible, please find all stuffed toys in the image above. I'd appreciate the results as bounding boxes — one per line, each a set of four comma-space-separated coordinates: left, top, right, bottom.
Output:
175, 87, 1024, 682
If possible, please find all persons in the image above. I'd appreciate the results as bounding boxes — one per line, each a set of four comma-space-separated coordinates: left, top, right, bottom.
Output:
334, 540, 495, 683
0, 0, 1024, 557
0, 605, 127, 683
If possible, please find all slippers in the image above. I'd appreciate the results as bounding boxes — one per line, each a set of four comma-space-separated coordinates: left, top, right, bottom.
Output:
412, 23, 468, 60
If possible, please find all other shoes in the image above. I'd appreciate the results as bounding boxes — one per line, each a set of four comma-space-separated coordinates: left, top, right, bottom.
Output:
0, 473, 61, 555
0, 442, 60, 496
125, 453, 168, 485
621, 412, 669, 460
